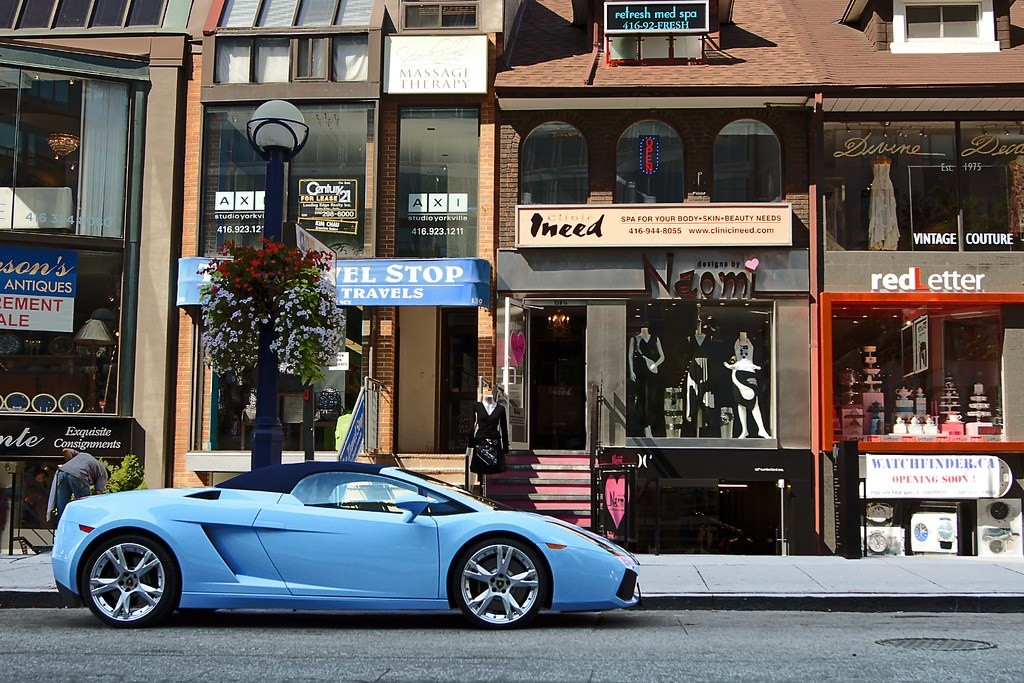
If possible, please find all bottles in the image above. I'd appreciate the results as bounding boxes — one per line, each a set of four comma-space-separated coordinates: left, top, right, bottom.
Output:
908, 417, 921, 434
894, 417, 906, 434
923, 418, 937, 435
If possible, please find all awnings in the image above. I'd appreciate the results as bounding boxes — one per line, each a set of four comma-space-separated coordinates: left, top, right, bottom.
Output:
175, 258, 492, 307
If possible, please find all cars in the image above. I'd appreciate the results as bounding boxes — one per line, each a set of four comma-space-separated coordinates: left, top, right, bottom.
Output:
50, 461, 640, 631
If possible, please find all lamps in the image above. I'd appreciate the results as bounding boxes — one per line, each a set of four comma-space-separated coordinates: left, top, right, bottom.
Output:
548, 308, 570, 338
73, 318, 116, 413
46, 133, 80, 160
845, 122, 1024, 138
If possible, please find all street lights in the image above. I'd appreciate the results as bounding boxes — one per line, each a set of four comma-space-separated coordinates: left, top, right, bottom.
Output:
247, 99, 309, 470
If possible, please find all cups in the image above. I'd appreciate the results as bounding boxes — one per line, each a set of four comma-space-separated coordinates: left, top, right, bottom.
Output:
949, 415, 962, 421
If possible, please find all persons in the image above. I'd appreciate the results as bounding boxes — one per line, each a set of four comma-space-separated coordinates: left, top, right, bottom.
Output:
629, 327, 664, 437
56, 449, 111, 526
867, 153, 901, 251
468, 393, 509, 474
681, 333, 718, 428
725, 332, 772, 439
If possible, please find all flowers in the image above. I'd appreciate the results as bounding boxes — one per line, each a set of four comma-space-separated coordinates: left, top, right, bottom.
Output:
196, 237, 347, 387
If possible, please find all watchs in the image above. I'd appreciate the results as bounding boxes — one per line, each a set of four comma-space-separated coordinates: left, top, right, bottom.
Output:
865, 502, 1020, 555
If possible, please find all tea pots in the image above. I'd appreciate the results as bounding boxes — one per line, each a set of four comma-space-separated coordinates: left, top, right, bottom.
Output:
895, 386, 913, 398
